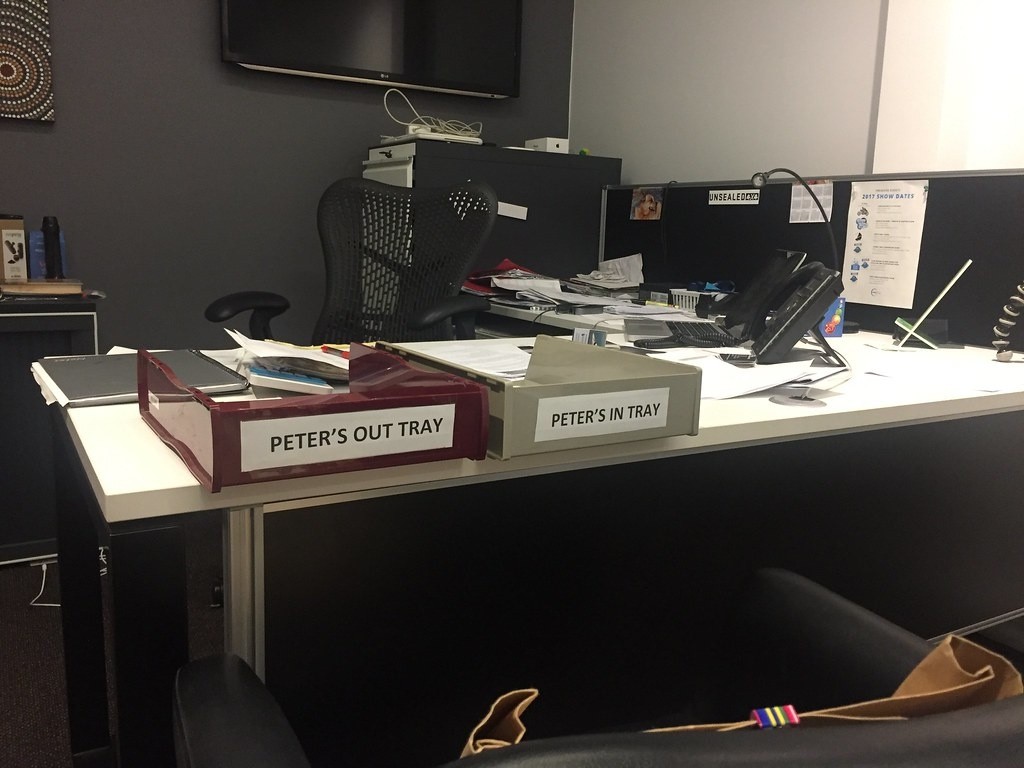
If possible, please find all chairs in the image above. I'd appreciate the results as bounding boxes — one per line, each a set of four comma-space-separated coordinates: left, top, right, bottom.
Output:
171, 563, 1024, 768
204, 175, 498, 345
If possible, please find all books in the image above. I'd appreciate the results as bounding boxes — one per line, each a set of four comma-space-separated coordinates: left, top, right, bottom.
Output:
31, 347, 252, 408
460, 259, 566, 295
0, 279, 83, 296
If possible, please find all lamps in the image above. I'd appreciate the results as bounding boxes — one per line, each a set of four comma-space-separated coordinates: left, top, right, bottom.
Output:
752, 168, 840, 270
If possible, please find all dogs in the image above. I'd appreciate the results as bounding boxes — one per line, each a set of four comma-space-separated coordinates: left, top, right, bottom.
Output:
633, 194, 662, 220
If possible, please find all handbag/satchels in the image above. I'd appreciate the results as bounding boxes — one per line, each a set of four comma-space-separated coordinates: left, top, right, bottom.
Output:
459, 634, 1024, 758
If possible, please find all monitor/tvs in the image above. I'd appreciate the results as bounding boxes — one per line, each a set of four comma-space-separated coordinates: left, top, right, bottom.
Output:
220, 0, 523, 99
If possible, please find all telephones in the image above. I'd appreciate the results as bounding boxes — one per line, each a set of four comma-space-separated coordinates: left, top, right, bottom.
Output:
740, 261, 843, 364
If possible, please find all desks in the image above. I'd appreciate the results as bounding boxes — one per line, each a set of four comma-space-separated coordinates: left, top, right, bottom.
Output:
0, 303, 96, 565
47, 302, 1024, 686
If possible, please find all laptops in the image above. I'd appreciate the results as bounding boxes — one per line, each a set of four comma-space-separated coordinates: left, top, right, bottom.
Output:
381, 132, 483, 146
624, 249, 806, 340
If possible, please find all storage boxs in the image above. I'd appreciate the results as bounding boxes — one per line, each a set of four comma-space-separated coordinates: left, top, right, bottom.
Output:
526, 137, 570, 154
